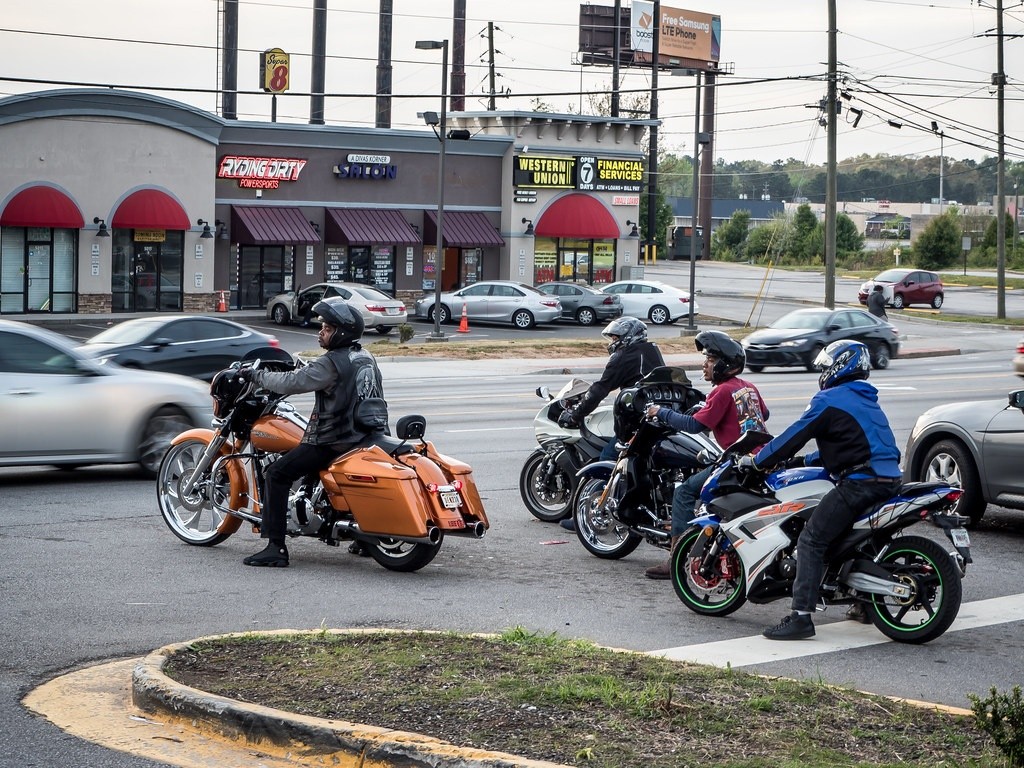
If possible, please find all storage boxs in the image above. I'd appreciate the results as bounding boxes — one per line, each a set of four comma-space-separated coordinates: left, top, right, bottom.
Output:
328, 445, 429, 538
414, 441, 490, 531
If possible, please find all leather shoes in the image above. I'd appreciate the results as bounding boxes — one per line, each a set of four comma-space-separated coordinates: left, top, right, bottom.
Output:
763, 611, 816, 639
244, 543, 291, 568
559, 517, 574, 530
846, 606, 872, 623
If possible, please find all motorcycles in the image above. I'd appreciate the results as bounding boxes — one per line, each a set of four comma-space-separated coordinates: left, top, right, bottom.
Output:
156, 348, 489, 571
520, 368, 974, 643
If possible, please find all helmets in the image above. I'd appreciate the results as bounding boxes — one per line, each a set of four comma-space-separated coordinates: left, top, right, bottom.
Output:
601, 316, 650, 351
312, 297, 365, 347
813, 337, 869, 390
694, 330, 748, 382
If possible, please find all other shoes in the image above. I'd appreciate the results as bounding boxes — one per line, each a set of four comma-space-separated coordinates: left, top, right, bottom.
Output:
645, 557, 672, 580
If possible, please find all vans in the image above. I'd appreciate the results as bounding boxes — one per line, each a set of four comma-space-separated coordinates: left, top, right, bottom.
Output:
564, 253, 610, 281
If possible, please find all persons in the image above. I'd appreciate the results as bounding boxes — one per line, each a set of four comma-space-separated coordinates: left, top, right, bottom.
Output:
643, 330, 770, 580
239, 300, 389, 567
866, 285, 891, 323
559, 316, 674, 529
737, 339, 903, 639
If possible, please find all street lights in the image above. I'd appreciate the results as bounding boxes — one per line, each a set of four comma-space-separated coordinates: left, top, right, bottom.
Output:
671, 68, 710, 336
414, 39, 449, 343
931, 121, 943, 217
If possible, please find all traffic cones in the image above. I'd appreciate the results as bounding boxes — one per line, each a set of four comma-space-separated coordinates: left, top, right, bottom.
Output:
456, 304, 471, 333
215, 290, 229, 312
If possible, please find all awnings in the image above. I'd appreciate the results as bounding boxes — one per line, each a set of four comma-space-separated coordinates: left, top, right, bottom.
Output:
230, 205, 320, 245
325, 208, 423, 247
424, 211, 505, 246
111, 189, 191, 230
0, 186, 84, 228
535, 193, 619, 238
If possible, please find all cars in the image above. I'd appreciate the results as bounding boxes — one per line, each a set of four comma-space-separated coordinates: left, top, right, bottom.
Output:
901, 339, 1024, 528
740, 307, 900, 373
858, 268, 944, 309
246, 270, 291, 305
537, 281, 624, 326
599, 280, 700, 325
112, 247, 181, 309
0, 319, 218, 480
415, 280, 563, 330
266, 284, 408, 335
45, 315, 279, 384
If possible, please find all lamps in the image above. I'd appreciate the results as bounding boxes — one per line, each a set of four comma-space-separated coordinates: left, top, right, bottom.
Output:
522, 218, 535, 235
626, 220, 638, 237
310, 221, 320, 235
94, 217, 110, 237
215, 220, 229, 240
410, 223, 420, 236
198, 219, 213, 239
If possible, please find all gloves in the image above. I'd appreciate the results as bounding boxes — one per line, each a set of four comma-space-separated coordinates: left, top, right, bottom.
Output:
233, 367, 259, 382
558, 411, 576, 428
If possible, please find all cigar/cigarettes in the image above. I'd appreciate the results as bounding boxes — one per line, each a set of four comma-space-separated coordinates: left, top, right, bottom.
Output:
700, 372, 706, 380
